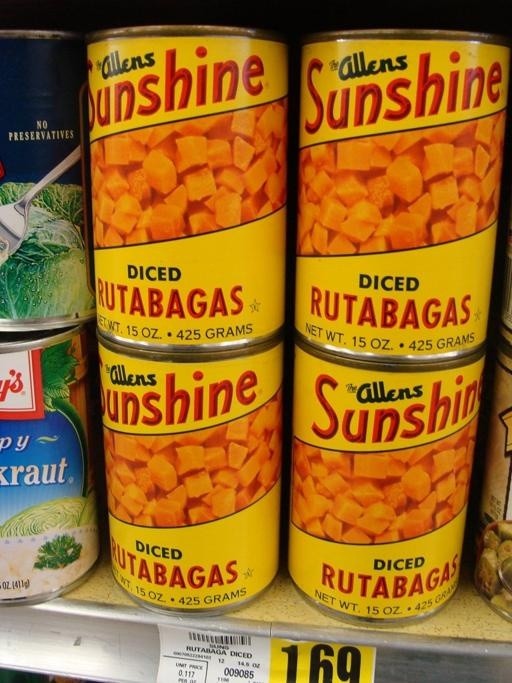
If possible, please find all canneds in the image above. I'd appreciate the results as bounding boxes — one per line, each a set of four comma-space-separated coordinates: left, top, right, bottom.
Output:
285, 29, 512, 625
469, 229, 512, 622
88, 26, 285, 617
1, 30, 96, 606
